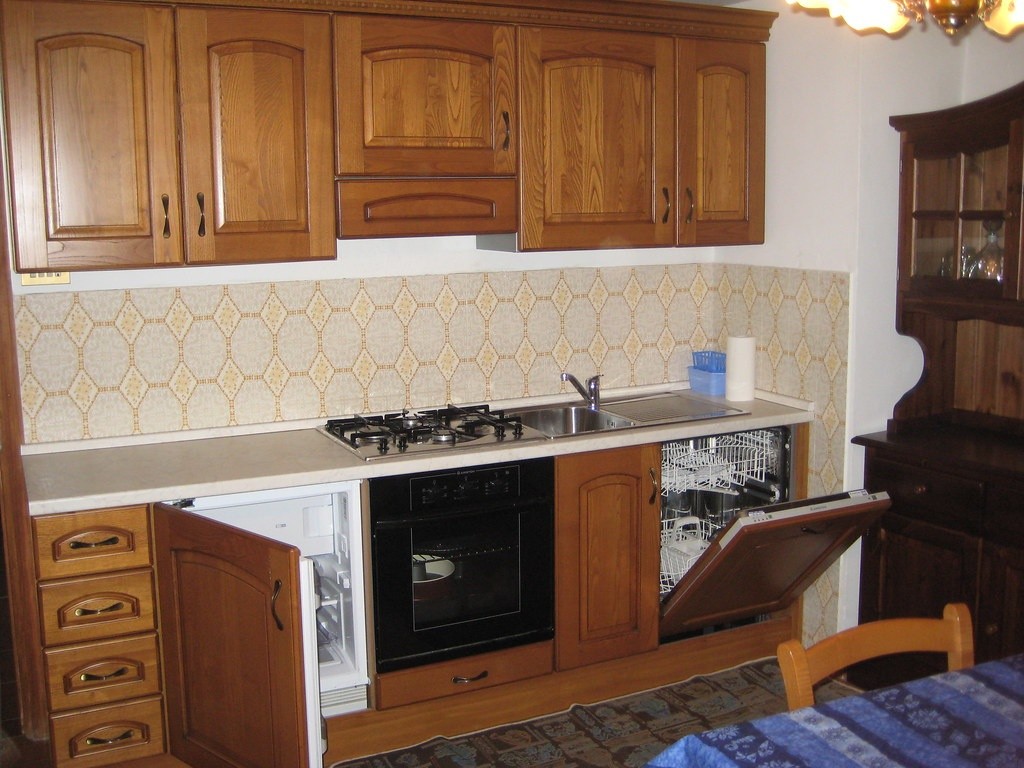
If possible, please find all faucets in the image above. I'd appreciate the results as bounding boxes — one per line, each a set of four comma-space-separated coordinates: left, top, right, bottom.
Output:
559, 370, 603, 408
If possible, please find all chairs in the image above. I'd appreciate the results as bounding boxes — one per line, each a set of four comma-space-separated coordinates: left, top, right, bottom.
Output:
777, 602, 973, 714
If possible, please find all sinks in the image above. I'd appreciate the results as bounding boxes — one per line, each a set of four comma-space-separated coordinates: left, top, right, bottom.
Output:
498, 402, 636, 440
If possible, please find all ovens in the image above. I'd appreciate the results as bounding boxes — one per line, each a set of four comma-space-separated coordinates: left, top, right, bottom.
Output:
368, 457, 555, 674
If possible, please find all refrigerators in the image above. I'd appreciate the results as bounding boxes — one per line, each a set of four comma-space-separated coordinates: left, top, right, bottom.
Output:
151, 478, 368, 768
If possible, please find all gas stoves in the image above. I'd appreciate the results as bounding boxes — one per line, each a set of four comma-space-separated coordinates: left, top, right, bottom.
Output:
315, 402, 548, 463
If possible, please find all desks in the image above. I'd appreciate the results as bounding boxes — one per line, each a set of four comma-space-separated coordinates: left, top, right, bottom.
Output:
644, 653, 1024, 768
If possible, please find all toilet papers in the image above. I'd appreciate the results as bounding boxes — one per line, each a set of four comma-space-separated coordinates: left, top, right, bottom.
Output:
723, 333, 757, 402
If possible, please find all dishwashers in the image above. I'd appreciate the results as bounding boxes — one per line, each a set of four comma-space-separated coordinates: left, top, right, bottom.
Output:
660, 425, 890, 642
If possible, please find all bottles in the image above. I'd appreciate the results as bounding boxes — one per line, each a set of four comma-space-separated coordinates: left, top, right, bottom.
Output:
968, 233, 1004, 280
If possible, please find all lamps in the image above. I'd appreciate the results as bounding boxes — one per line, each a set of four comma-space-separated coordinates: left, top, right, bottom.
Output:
786, 0, 1024, 36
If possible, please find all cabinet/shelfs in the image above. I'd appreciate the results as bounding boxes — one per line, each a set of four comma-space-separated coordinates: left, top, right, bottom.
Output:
29, 502, 169, 768
886, 80, 1024, 442
0, 0, 338, 273
336, 0, 520, 241
475, 0, 780, 253
554, 442, 660, 673
154, 479, 376, 768
847, 430, 1024, 693
